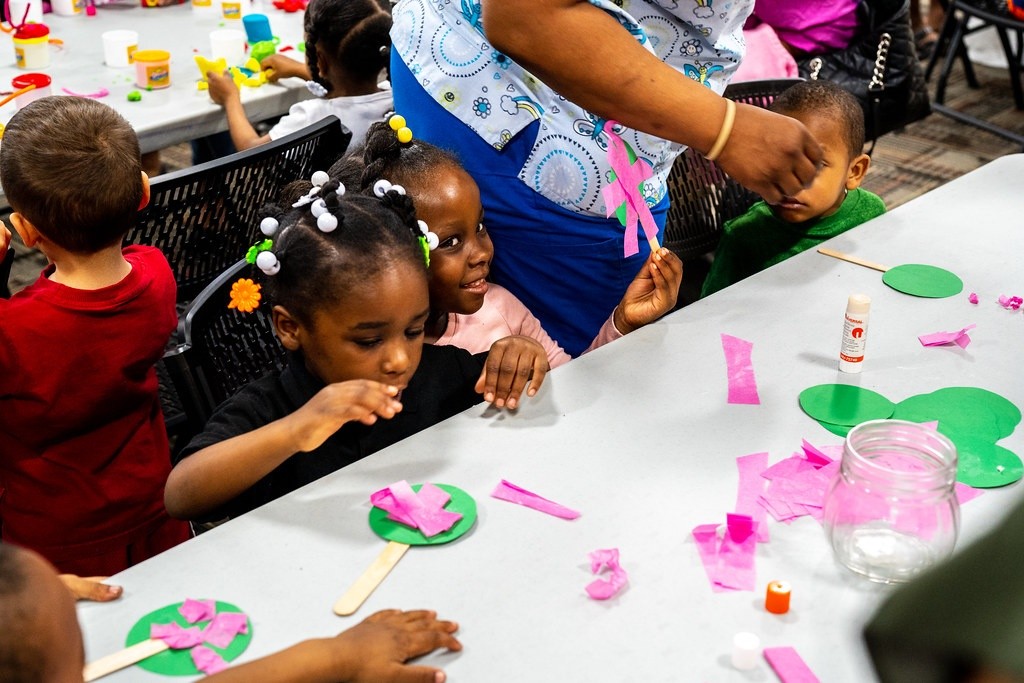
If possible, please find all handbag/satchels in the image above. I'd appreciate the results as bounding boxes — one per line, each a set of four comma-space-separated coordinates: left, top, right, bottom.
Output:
782, 0, 932, 145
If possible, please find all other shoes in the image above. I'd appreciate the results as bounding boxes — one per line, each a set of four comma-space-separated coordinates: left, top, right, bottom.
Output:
915, 28, 938, 61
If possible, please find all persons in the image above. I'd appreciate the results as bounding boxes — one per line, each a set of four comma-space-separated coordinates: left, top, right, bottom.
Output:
163, 170, 549, 522
178, 0, 396, 237
698, 79, 888, 302
0, 95, 190, 578
0, 542, 463, 683
389, 0, 827, 361
328, 111, 684, 370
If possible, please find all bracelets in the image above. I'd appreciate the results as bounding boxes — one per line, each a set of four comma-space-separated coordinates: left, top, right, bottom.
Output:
702, 96, 737, 162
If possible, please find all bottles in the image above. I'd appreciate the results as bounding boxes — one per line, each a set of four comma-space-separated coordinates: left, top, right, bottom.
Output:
1, 0, 308, 112
824, 418, 963, 585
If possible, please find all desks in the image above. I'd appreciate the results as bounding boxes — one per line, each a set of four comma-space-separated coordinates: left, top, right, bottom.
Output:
0, 0, 388, 194
73, 153, 1023, 683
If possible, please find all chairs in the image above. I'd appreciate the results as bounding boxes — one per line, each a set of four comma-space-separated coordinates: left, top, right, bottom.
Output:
0, 0, 1024, 538
